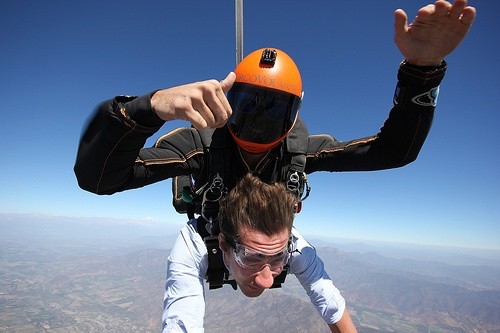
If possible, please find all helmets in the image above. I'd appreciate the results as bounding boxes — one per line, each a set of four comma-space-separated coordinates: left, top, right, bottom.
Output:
224, 47, 304, 153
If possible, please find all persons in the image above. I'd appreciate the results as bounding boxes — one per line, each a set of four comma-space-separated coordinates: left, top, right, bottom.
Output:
73, 0, 476, 214
162, 172, 362, 333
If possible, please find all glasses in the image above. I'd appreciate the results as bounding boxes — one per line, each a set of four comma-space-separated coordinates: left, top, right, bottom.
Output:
232, 242, 290, 271
